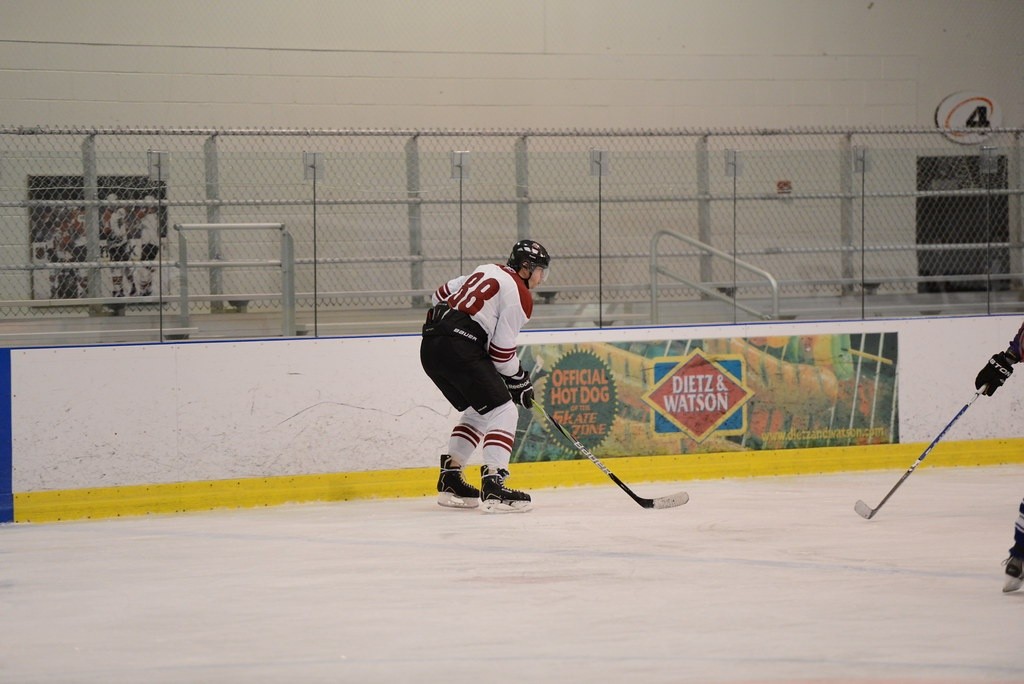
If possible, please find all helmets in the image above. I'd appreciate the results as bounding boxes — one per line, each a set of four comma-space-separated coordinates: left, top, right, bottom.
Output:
506, 239, 551, 273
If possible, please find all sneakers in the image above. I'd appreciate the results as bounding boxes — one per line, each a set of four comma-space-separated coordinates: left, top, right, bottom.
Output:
1001, 554, 1024, 592
437, 455, 480, 508
480, 465, 533, 514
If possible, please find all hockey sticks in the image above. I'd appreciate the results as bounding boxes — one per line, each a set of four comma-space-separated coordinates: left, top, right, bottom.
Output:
531, 399, 689, 509
853, 383, 986, 519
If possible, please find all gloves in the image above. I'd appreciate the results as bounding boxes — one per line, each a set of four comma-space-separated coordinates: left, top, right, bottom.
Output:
976, 352, 1013, 396
504, 371, 535, 410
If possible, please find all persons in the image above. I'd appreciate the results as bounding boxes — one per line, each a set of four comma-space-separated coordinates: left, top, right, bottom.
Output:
51, 193, 159, 305
975, 321, 1024, 593
420, 239, 551, 514
